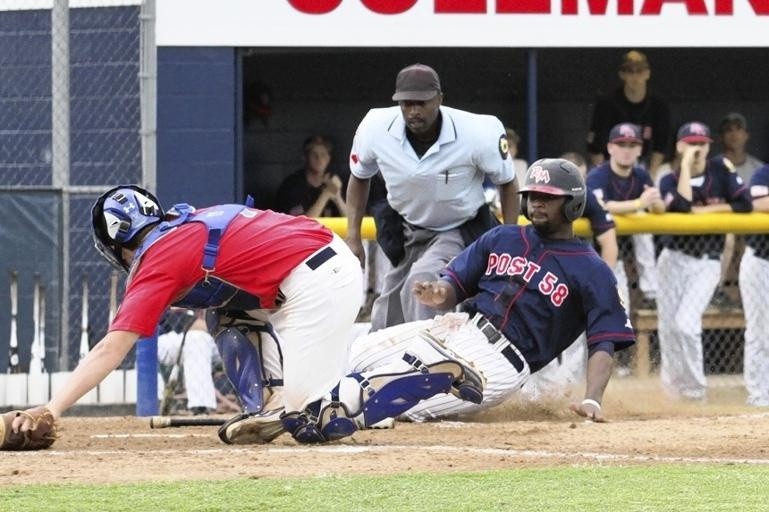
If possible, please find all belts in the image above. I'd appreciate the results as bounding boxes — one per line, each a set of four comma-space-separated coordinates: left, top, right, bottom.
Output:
269, 247, 337, 305
465, 304, 525, 374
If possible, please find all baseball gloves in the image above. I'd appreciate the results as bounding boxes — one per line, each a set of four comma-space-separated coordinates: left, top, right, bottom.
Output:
1, 405, 58, 450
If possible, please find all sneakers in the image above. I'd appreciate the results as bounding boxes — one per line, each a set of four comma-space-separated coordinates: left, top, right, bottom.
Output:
218, 407, 288, 445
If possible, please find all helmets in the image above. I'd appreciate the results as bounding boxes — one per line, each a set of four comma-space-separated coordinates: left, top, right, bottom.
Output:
516, 158, 588, 222
91, 185, 163, 243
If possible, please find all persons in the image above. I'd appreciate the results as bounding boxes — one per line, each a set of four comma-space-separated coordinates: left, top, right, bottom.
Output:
156, 302, 223, 415
348, 159, 639, 422
345, 62, 520, 332
269, 134, 348, 220
484, 49, 769, 407
1, 184, 487, 453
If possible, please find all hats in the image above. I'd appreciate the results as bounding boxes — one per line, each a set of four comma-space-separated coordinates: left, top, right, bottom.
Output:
717, 112, 748, 131
504, 128, 520, 143
609, 122, 644, 144
301, 134, 334, 152
620, 50, 648, 67
678, 120, 714, 145
391, 64, 442, 101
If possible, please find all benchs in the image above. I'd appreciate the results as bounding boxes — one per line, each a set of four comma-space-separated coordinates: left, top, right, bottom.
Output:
630, 306, 746, 379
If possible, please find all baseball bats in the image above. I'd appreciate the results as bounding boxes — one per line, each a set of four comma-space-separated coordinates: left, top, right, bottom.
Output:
150, 416, 227, 429
